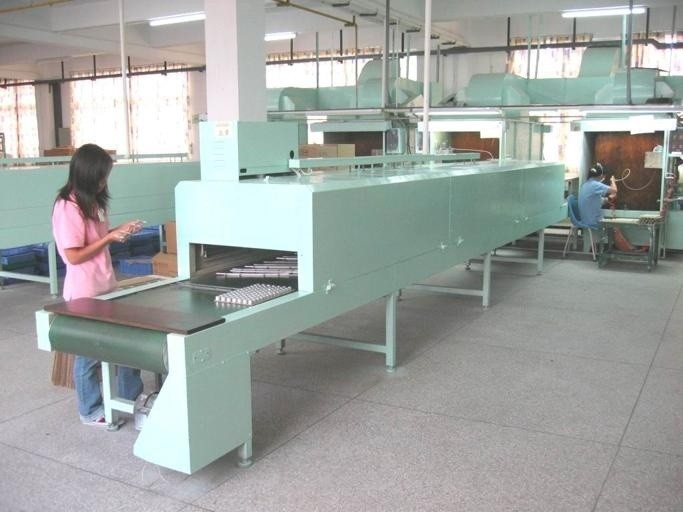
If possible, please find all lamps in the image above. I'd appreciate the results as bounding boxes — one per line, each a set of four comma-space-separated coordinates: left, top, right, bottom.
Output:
560, 4, 645, 18
405, 105, 500, 120
263, 29, 296, 42
301, 107, 384, 118
577, 105, 683, 117
147, 10, 206, 28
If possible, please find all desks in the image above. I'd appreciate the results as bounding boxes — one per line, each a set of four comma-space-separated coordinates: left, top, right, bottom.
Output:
596, 214, 669, 275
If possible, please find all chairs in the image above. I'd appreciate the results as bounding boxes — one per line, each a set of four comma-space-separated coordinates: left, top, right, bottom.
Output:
562, 192, 602, 261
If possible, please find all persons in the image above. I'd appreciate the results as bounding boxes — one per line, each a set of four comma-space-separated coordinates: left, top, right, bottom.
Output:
49, 143, 146, 428
577, 161, 617, 230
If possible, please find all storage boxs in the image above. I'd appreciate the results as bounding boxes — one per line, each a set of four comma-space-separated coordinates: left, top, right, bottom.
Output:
331, 143, 357, 171
164, 220, 178, 253
298, 143, 337, 173
151, 251, 180, 276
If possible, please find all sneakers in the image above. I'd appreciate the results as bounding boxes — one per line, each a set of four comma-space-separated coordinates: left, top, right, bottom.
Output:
631, 245, 650, 253
79, 412, 126, 426
